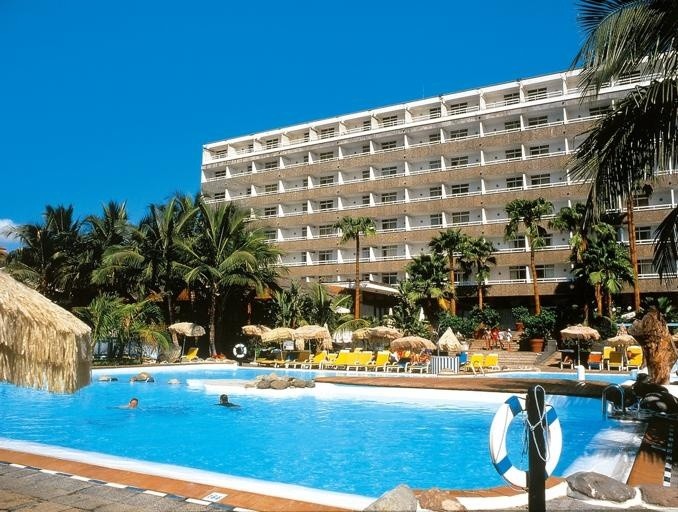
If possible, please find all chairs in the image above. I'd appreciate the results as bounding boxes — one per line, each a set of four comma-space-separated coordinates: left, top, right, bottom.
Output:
486, 354, 501, 372
588, 352, 605, 371
180, 346, 199, 362
461, 353, 486, 375
255, 349, 430, 373
560, 351, 575, 370
626, 346, 642, 371
607, 351, 623, 370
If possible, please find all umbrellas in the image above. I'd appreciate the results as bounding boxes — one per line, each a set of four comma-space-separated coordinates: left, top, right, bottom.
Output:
559, 324, 601, 365
0, 270, 92, 394
169, 320, 206, 356
261, 325, 462, 370
606, 334, 637, 352
241, 323, 271, 362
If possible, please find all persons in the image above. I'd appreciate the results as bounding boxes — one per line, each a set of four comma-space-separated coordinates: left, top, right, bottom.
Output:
214, 393, 240, 407
481, 327, 493, 350
497, 329, 505, 350
504, 328, 513, 351
127, 397, 138, 409
491, 327, 499, 349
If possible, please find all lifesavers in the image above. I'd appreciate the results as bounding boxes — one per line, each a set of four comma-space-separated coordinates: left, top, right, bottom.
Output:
489, 394, 563, 487
233, 343, 248, 358
